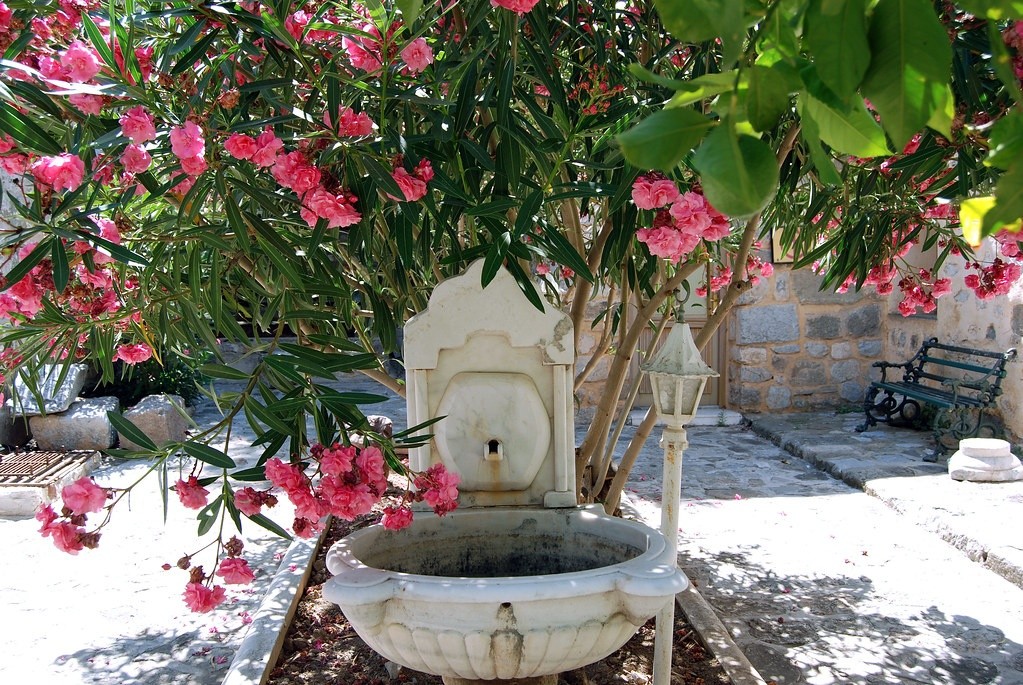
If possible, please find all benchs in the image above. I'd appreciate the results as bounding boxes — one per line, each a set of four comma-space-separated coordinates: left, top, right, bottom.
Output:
855, 336, 1018, 464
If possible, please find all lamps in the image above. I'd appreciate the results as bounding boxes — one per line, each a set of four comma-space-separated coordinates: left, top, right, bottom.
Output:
641, 302, 721, 429
